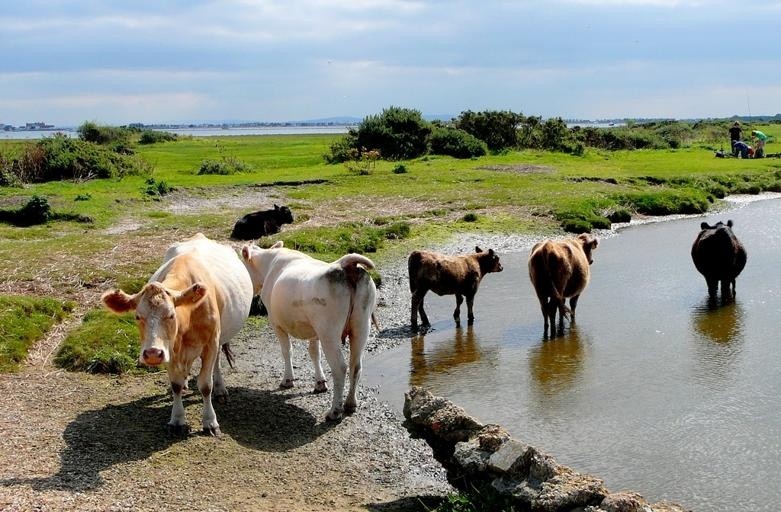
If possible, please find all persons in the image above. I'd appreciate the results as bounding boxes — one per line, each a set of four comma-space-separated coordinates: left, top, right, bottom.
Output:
731, 140, 751, 158
751, 131, 768, 158
729, 121, 742, 153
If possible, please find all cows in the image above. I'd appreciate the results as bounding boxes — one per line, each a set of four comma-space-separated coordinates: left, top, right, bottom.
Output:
99, 231, 255, 437
690, 219, 748, 302
407, 245, 504, 325
239, 238, 381, 423
527, 231, 598, 327
230, 203, 294, 240
